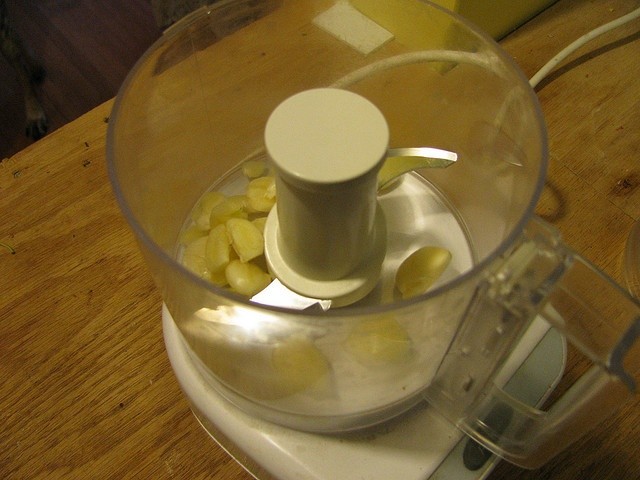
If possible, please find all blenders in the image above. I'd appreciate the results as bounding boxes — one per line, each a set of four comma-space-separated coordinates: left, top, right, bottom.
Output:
106, 0, 640, 478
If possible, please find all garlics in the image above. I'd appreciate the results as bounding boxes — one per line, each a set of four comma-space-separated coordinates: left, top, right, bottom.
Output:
175, 160, 456, 403
